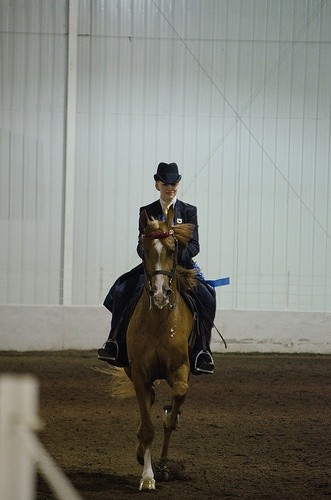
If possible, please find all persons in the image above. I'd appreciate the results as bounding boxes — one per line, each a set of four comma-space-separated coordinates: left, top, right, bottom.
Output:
97, 162, 217, 371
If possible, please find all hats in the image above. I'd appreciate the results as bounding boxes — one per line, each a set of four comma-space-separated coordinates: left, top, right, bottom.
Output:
154, 162, 182, 184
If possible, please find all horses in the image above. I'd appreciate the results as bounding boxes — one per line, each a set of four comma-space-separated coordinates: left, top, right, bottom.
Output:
85, 203, 196, 492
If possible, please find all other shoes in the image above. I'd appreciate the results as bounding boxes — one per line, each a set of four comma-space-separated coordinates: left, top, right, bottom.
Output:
190, 357, 216, 375
97, 347, 126, 368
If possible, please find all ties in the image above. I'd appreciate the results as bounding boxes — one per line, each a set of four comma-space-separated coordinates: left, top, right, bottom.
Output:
163, 203, 170, 217
